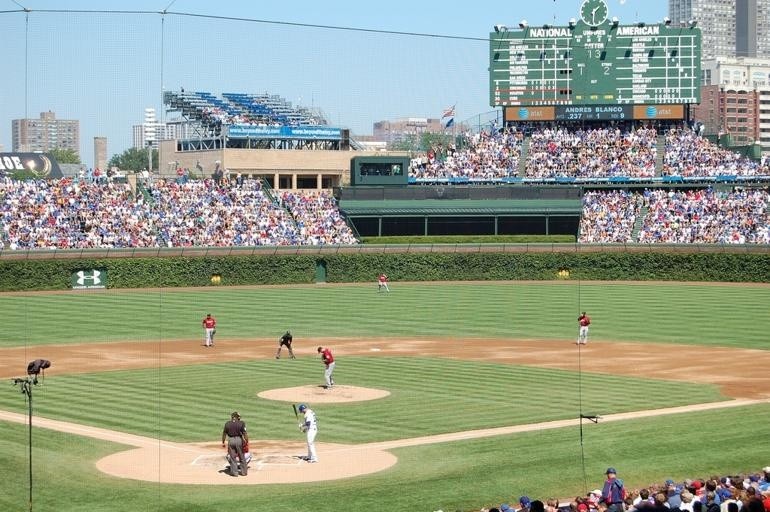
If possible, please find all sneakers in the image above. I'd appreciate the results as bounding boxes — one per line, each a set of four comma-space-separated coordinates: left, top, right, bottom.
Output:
304, 457, 317, 462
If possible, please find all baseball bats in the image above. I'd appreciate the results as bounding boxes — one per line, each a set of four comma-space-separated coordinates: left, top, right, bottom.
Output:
292, 404, 303, 432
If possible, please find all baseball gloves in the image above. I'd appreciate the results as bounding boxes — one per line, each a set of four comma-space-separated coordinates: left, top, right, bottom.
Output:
213, 328, 216, 334
242, 440, 249, 452
322, 354, 326, 363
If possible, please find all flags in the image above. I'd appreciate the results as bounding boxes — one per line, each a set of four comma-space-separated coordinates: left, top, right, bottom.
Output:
439, 105, 456, 129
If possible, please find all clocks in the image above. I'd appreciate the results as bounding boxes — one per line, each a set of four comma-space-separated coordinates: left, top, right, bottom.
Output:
579, 0, 609, 28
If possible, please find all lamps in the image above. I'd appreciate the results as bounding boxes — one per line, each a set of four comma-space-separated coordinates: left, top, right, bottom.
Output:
494, 25, 508, 33
688, 20, 697, 28
569, 19, 576, 29
613, 17, 620, 27
519, 20, 527, 32
664, 16, 671, 25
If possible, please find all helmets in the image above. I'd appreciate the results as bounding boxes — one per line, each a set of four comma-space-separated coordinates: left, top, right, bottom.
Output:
44, 361, 50, 368
299, 405, 306, 413
286, 330, 290, 335
231, 412, 240, 420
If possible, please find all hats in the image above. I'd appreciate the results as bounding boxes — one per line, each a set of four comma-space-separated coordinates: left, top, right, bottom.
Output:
603, 468, 616, 474
590, 489, 602, 496
762, 466, 770, 474
665, 480, 674, 486
577, 504, 587, 511
501, 496, 531, 512
318, 347, 322, 353
691, 480, 701, 488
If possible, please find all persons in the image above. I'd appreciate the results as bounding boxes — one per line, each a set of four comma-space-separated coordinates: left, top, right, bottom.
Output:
276, 330, 295, 360
575, 184, 770, 247
222, 411, 248, 476
576, 312, 591, 344
377, 273, 390, 292
202, 312, 217, 347
225, 433, 251, 465
317, 346, 336, 388
391, 119, 769, 187
480, 466, 770, 511
298, 404, 318, 463
1, 159, 360, 252
203, 107, 320, 127
